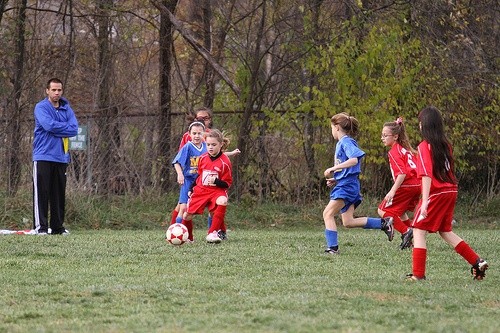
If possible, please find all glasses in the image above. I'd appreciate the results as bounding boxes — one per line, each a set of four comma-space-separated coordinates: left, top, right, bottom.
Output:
197, 117, 211, 121
381, 134, 397, 138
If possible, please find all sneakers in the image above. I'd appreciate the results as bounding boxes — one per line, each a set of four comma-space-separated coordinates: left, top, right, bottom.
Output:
380, 216, 394, 242
471, 259, 488, 279
186, 239, 194, 245
205, 228, 222, 243
401, 227, 414, 249
324, 248, 338, 255
406, 273, 425, 282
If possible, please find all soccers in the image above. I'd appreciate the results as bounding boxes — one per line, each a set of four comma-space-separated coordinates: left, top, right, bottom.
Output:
166, 222, 189, 245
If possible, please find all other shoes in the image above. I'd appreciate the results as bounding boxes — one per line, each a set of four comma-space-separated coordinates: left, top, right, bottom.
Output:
52, 229, 69, 235
39, 231, 47, 235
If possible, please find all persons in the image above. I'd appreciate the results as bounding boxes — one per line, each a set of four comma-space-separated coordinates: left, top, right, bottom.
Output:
169, 106, 241, 245
377, 116, 422, 250
322, 112, 394, 255
404, 104, 489, 282
31, 78, 78, 235
